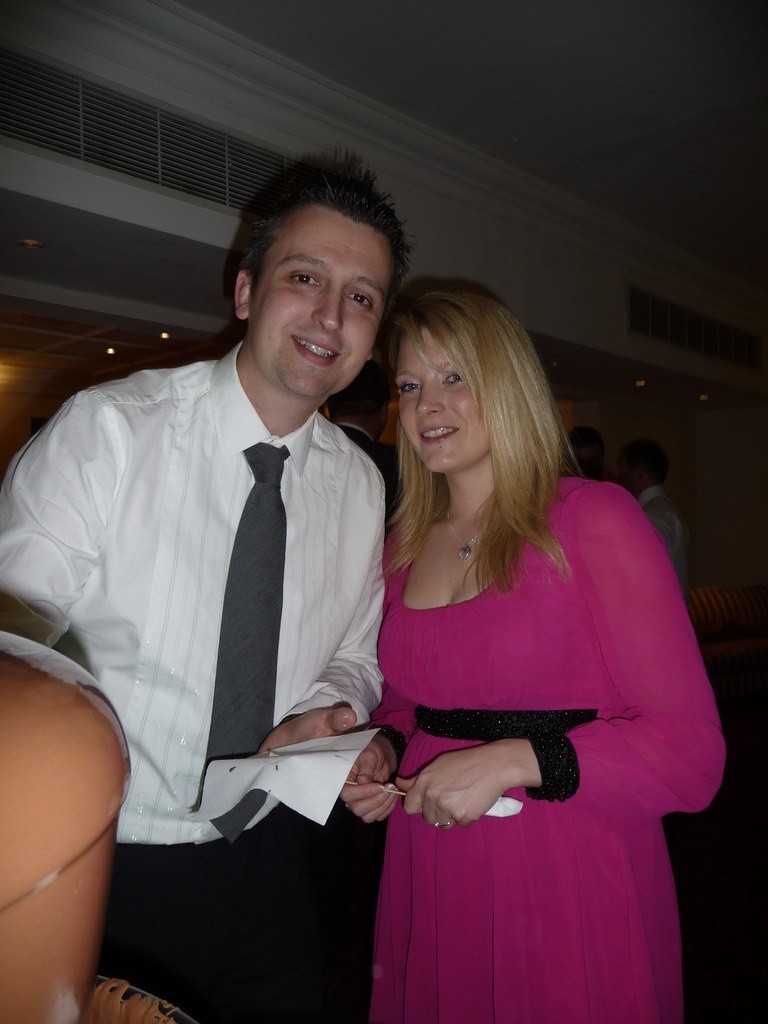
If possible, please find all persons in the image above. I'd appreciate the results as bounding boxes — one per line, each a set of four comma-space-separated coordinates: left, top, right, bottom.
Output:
0, 152, 403, 1024
341, 286, 727, 1024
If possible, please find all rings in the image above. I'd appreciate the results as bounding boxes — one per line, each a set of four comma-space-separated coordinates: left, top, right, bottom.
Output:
434, 821, 453, 828
344, 803, 353, 812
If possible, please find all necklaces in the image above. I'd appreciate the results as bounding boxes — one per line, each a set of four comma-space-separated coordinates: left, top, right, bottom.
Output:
446, 510, 489, 560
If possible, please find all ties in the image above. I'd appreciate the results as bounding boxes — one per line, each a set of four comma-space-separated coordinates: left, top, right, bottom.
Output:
191, 443, 292, 848
